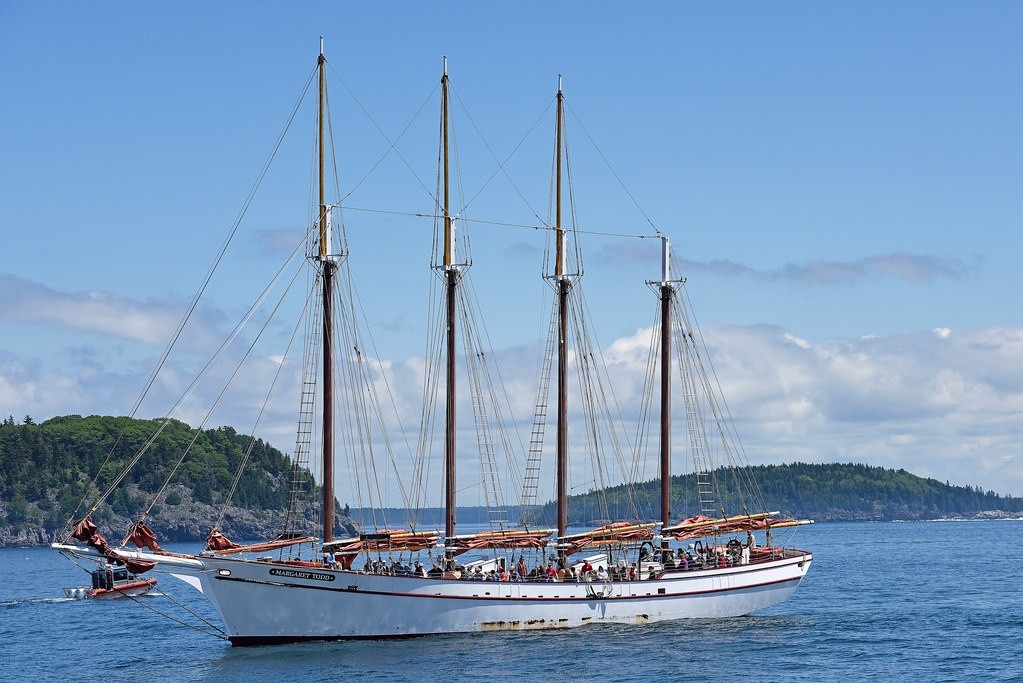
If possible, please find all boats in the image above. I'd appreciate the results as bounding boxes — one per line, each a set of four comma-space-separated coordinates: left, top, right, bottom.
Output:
60, 563, 158, 600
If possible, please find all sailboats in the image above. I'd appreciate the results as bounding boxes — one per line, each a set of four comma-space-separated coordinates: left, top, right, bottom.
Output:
45, 32, 817, 648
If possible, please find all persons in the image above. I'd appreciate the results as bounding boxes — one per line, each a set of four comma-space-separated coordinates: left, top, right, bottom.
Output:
581, 560, 636, 581
364, 557, 443, 579
563, 567, 578, 582
646, 566, 656, 580
638, 546, 662, 563
455, 555, 528, 582
326, 546, 336, 570
665, 548, 734, 570
525, 563, 559, 582
746, 530, 756, 550
